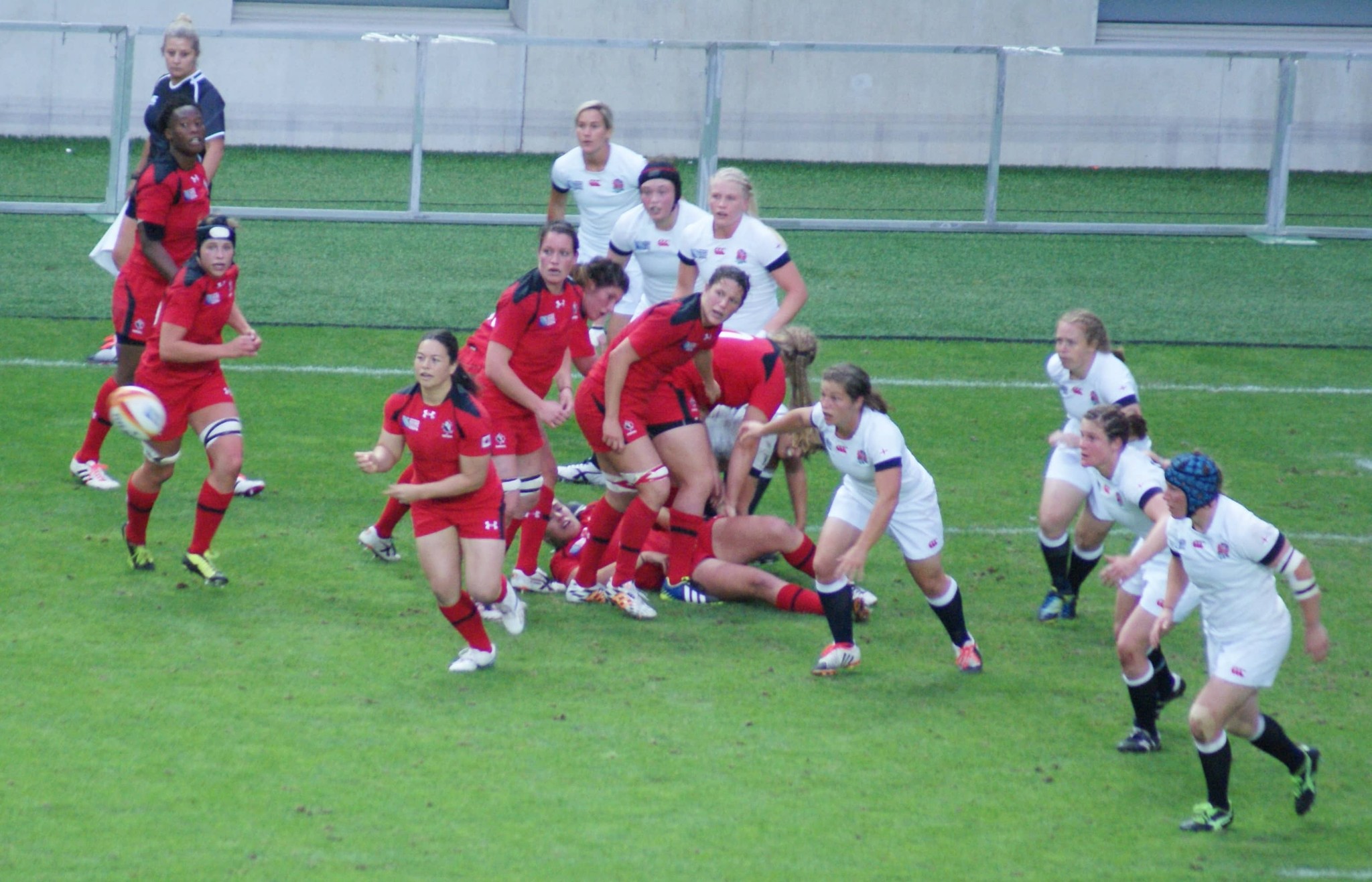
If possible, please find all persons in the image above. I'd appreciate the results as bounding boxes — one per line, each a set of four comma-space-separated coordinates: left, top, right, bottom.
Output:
357, 255, 632, 593
469, 220, 575, 621
68, 98, 265, 500
736, 362, 982, 677
1078, 402, 1203, 754
1149, 448, 1330, 832
566, 266, 751, 619
567, 326, 818, 523
546, 99, 647, 348
542, 497, 878, 621
660, 402, 823, 531
121, 213, 263, 589
607, 160, 713, 308
676, 166, 807, 339
352, 328, 526, 672
89, 12, 226, 364
1035, 310, 1143, 622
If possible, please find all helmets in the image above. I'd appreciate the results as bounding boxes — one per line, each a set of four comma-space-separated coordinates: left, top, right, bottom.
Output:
1164, 452, 1220, 512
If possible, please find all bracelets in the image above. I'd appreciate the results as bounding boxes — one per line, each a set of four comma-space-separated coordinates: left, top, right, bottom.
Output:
1157, 598, 1174, 611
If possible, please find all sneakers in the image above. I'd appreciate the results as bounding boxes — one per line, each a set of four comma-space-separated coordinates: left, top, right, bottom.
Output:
952, 629, 983, 672
121, 522, 155, 570
1116, 726, 1162, 753
565, 579, 616, 606
448, 643, 496, 673
1040, 586, 1060, 620
180, 551, 228, 586
659, 575, 726, 605
357, 526, 400, 561
851, 597, 868, 621
849, 580, 878, 606
812, 639, 861, 675
556, 459, 606, 486
567, 499, 587, 521
1179, 799, 1234, 831
69, 456, 120, 489
1059, 595, 1077, 619
604, 576, 657, 619
233, 475, 265, 498
496, 593, 526, 636
1291, 744, 1321, 816
1132, 671, 1187, 726
510, 567, 567, 594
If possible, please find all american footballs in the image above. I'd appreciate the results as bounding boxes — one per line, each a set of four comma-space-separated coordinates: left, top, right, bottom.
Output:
106, 385, 167, 441
